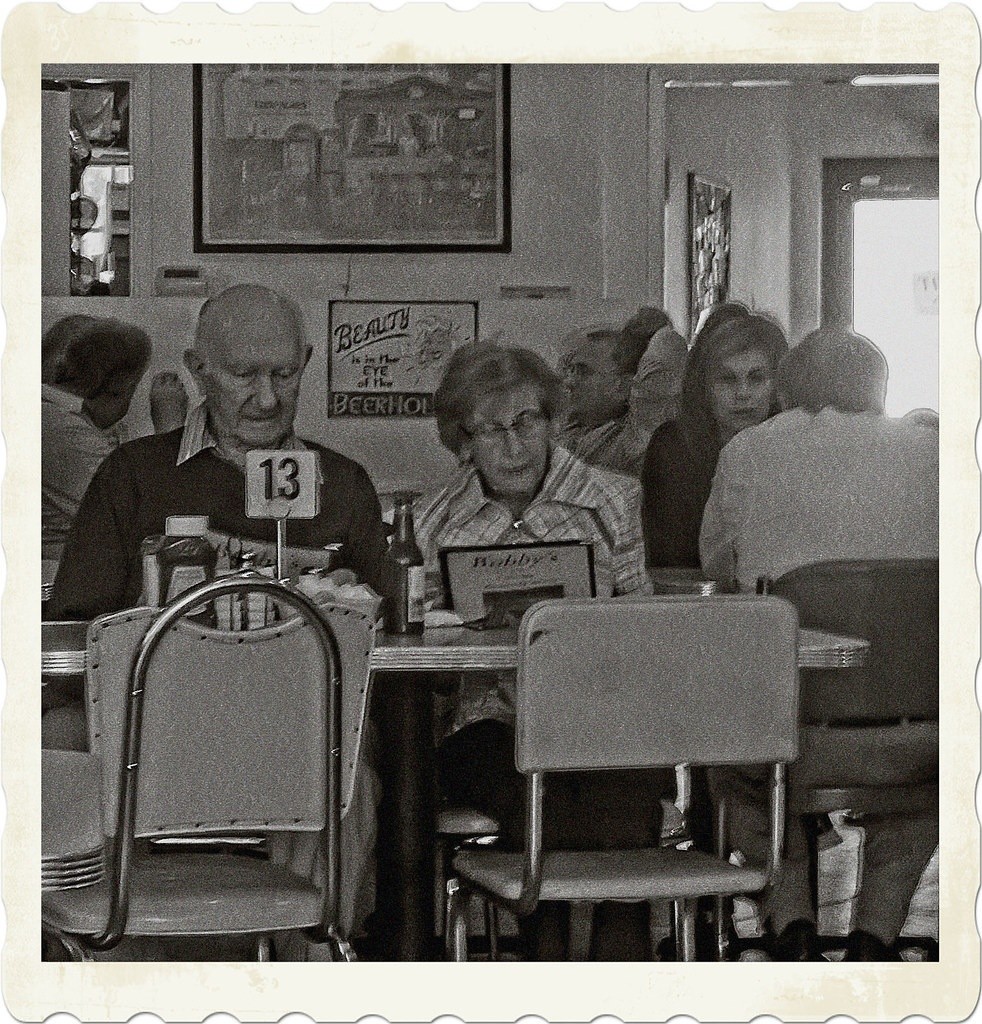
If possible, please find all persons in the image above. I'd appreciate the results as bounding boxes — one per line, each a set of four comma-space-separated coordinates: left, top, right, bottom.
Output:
44, 310, 194, 620
641, 308, 837, 963
553, 306, 691, 473
44, 286, 404, 962
383, 340, 663, 962
699, 332, 941, 961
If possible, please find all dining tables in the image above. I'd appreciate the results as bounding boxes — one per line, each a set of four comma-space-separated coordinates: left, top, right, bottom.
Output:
41, 619, 873, 963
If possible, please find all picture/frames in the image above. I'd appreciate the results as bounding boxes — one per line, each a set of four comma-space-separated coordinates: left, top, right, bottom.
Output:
437, 537, 597, 631
328, 300, 478, 419
191, 64, 513, 252
687, 169, 732, 346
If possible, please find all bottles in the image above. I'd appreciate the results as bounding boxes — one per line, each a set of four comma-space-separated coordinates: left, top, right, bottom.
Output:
378, 494, 427, 634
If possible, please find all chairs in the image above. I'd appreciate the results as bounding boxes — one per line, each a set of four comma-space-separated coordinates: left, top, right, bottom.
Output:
42, 570, 375, 961
434, 558, 938, 961
